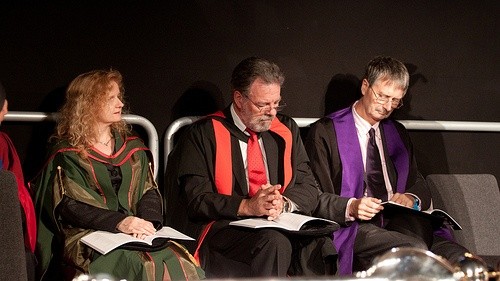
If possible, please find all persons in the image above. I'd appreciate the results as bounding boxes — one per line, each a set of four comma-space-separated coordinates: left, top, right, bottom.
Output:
166, 56, 339, 280
35, 69, 206, 281
0, 84, 38, 255
302, 56, 471, 271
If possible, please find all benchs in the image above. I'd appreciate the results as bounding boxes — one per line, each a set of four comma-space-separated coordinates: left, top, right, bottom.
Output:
0, 112, 500, 281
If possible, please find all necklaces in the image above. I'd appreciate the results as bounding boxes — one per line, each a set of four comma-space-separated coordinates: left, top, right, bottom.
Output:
97, 137, 114, 148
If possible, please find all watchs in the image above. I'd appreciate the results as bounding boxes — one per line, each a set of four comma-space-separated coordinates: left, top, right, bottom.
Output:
283, 197, 290, 212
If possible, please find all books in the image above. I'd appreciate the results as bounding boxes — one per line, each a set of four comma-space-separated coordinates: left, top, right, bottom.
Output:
227, 210, 339, 233
360, 201, 463, 232
78, 223, 197, 257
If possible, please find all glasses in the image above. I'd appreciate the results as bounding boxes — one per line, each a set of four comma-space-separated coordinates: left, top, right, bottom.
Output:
243, 93, 288, 113
371, 87, 403, 110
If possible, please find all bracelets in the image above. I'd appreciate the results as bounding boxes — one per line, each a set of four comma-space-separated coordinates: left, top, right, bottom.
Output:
414, 196, 419, 211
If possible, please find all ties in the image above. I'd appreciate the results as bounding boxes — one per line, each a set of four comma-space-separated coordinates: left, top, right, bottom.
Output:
365, 128, 389, 228
244, 127, 267, 197
